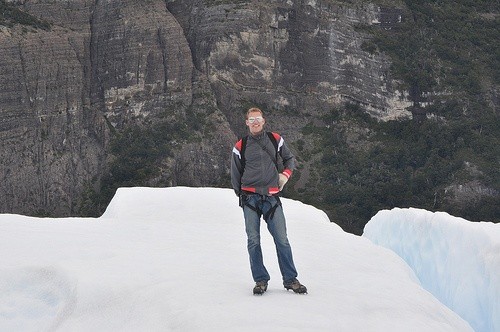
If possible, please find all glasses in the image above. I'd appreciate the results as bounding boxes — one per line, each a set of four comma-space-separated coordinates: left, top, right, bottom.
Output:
247, 116, 263, 123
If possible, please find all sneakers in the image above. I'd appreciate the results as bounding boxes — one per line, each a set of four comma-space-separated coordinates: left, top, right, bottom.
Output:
252, 280, 268, 294
283, 278, 307, 294
